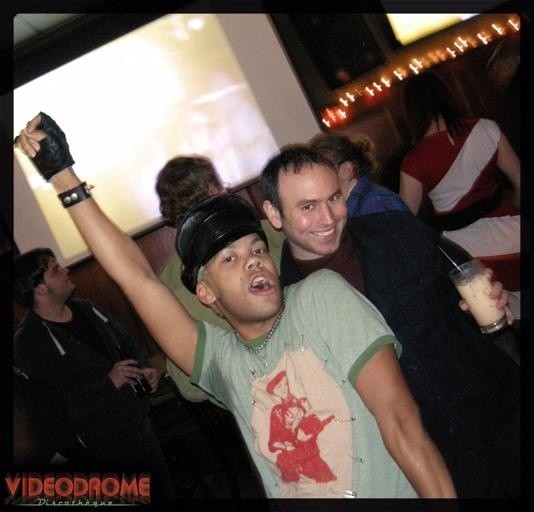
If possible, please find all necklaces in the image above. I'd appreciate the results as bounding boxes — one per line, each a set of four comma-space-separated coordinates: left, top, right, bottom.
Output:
233, 298, 286, 353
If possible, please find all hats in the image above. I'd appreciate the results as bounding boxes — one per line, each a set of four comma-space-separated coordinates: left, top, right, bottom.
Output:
175, 193, 270, 294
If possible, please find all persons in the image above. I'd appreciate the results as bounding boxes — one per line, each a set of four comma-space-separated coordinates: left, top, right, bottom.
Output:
149, 155, 287, 415
13, 112, 461, 498
398, 70, 520, 330
11, 246, 178, 501
257, 143, 516, 497
306, 129, 413, 217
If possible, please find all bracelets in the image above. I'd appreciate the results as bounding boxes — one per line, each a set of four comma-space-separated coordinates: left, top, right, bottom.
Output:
57, 182, 92, 208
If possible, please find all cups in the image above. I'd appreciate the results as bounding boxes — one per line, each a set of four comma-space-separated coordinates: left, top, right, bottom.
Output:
448, 259, 510, 335
129, 373, 151, 396
